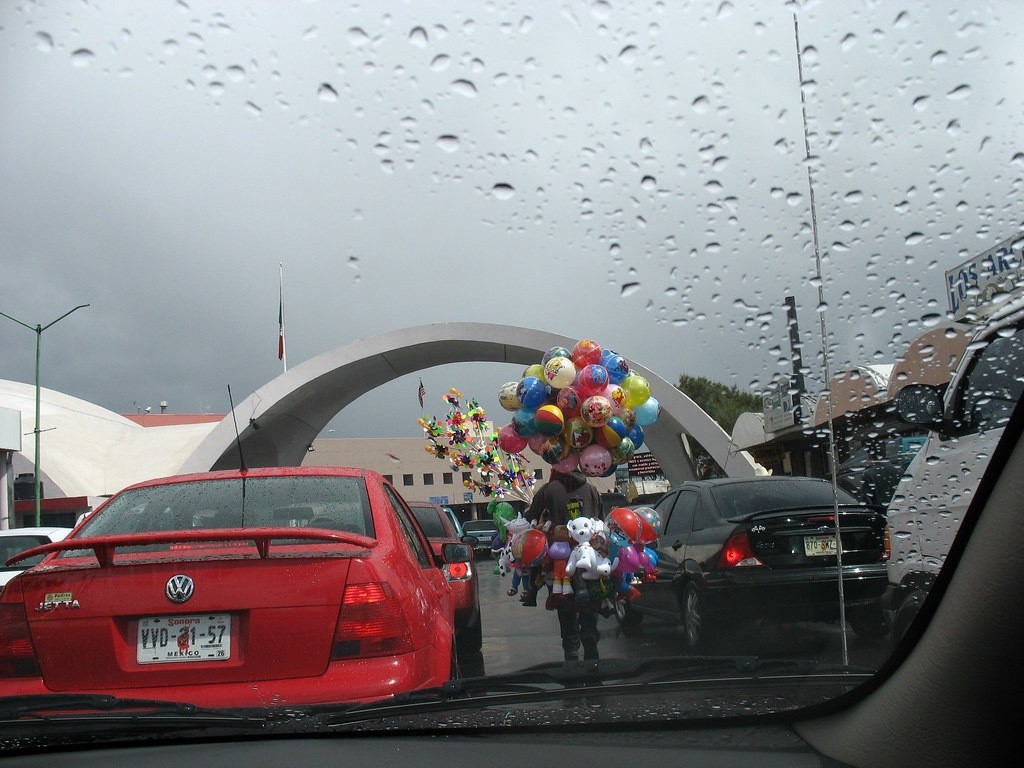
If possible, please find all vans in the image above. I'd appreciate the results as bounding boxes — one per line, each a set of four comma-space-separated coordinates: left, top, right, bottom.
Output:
884, 290, 1024, 648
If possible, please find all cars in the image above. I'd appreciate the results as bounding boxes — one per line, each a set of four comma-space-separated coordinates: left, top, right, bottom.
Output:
609, 476, 891, 650
0, 468, 472, 707
1, 528, 74, 586
461, 520, 497, 553
405, 500, 482, 652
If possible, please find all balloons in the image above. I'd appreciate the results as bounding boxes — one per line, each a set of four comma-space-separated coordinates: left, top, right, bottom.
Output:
602, 506, 663, 547
511, 529, 549, 567
497, 340, 661, 477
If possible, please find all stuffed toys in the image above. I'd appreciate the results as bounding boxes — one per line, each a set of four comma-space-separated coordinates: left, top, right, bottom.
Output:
547, 517, 620, 595
491, 512, 537, 577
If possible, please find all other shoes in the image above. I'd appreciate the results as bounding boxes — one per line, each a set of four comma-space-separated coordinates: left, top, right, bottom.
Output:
582, 635, 600, 660
564, 650, 580, 661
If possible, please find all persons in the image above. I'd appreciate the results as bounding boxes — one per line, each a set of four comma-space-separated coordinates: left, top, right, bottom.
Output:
491, 529, 506, 576
506, 568, 555, 610
527, 466, 604, 661
